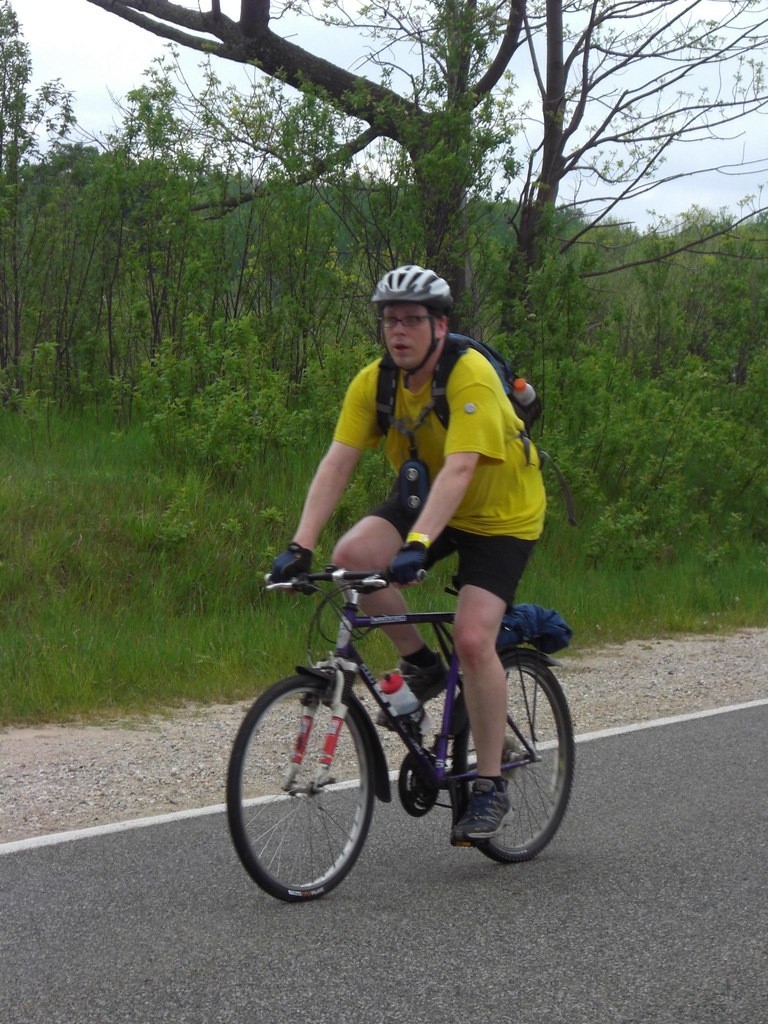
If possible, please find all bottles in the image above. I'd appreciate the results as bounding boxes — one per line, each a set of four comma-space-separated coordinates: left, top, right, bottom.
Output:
382, 673, 432, 737
511, 377, 537, 406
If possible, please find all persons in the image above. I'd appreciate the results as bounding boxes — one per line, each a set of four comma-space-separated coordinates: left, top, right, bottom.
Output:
272, 265, 547, 839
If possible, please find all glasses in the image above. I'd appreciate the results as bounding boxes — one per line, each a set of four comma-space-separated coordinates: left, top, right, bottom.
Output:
377, 315, 432, 328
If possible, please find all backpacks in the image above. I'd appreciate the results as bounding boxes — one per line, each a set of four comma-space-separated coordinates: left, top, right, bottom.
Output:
376, 333, 543, 448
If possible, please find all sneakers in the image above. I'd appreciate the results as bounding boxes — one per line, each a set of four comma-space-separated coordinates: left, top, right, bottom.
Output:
451, 779, 514, 838
376, 652, 449, 726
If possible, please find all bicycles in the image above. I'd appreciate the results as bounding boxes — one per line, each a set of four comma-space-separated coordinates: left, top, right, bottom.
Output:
225, 568, 576, 903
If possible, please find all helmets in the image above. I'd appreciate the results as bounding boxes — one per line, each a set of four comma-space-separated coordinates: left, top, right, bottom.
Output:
372, 265, 453, 308
